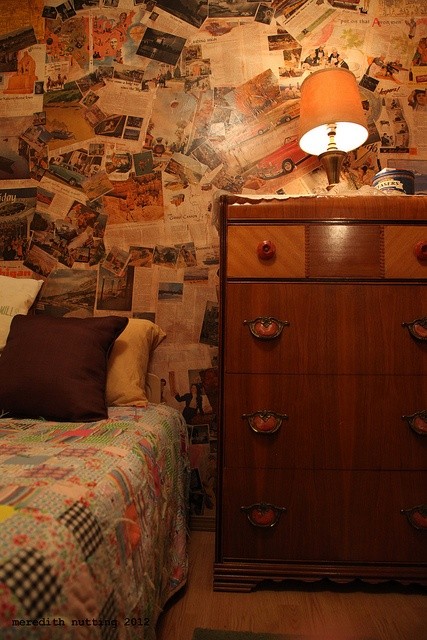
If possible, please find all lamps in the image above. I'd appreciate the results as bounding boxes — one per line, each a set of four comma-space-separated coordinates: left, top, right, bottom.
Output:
300, 69, 368, 195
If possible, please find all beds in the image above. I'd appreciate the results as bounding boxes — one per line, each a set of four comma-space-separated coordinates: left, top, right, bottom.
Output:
1, 373, 190, 639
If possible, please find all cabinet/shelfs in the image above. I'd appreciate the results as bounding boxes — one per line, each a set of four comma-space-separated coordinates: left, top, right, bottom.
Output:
214, 194, 427, 592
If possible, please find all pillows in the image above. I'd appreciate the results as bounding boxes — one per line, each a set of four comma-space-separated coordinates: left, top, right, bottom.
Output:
0, 314, 128, 422
107, 319, 167, 407
0, 275, 45, 348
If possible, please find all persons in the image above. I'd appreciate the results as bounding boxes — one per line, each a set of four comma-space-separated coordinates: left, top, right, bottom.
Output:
179, 246, 189, 262
120, 23, 150, 67
47, 76, 51, 86
303, 46, 324, 66
386, 58, 401, 80
160, 379, 169, 404
83, 92, 96, 107
380, 132, 394, 146
407, 89, 426, 111
365, 54, 386, 78
0, 203, 122, 272
327, 51, 348, 70
174, 384, 204, 424
404, 17, 416, 39
30, 153, 47, 172
57, 74, 61, 86
